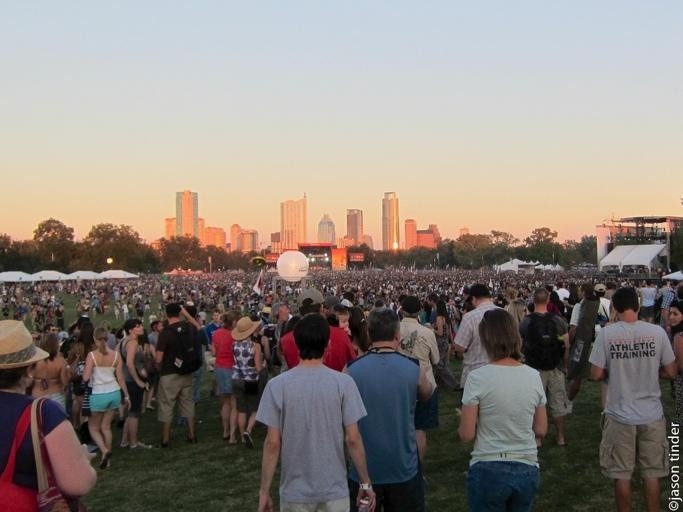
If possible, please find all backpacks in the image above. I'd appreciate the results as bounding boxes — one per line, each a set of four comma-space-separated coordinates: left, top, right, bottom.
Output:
524, 312, 560, 370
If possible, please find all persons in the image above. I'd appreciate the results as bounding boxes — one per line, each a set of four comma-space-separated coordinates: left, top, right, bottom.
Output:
0, 264, 681, 509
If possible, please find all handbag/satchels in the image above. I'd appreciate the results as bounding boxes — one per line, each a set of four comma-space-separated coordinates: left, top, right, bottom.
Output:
0, 477, 87, 512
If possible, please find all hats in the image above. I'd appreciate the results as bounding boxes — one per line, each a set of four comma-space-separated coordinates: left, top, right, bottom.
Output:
594, 283, 606, 292
0, 320, 50, 369
296, 288, 325, 308
231, 316, 262, 341
